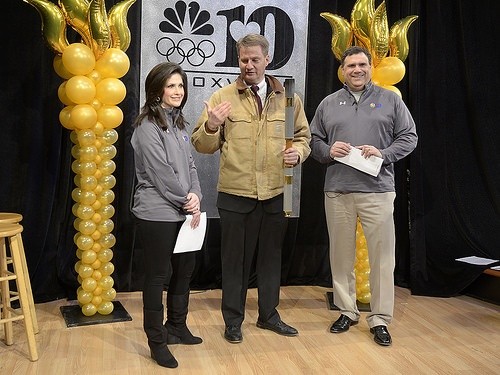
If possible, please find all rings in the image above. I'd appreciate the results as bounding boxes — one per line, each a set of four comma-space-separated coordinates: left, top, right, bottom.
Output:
368, 148, 371, 151
196, 206, 199, 210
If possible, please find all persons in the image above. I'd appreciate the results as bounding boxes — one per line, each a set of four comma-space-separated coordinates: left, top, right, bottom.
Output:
311, 47, 418, 346
131, 62, 204, 369
191, 34, 311, 341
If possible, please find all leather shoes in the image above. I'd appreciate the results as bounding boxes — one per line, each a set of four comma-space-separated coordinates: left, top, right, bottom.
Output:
224, 325, 243, 343
256, 320, 298, 336
370, 325, 392, 346
330, 314, 358, 333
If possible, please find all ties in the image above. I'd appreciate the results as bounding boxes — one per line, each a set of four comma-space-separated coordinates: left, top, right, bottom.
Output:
250, 85, 262, 118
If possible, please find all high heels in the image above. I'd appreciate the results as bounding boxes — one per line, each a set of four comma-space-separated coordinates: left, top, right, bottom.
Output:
148, 342, 178, 368
165, 322, 203, 344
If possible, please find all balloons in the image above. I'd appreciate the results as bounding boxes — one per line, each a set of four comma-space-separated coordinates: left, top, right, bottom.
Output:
338, 56, 406, 304
54, 43, 130, 318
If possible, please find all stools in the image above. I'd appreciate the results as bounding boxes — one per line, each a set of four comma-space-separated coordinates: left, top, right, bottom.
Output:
0, 221, 39, 362
0, 212, 39, 334
